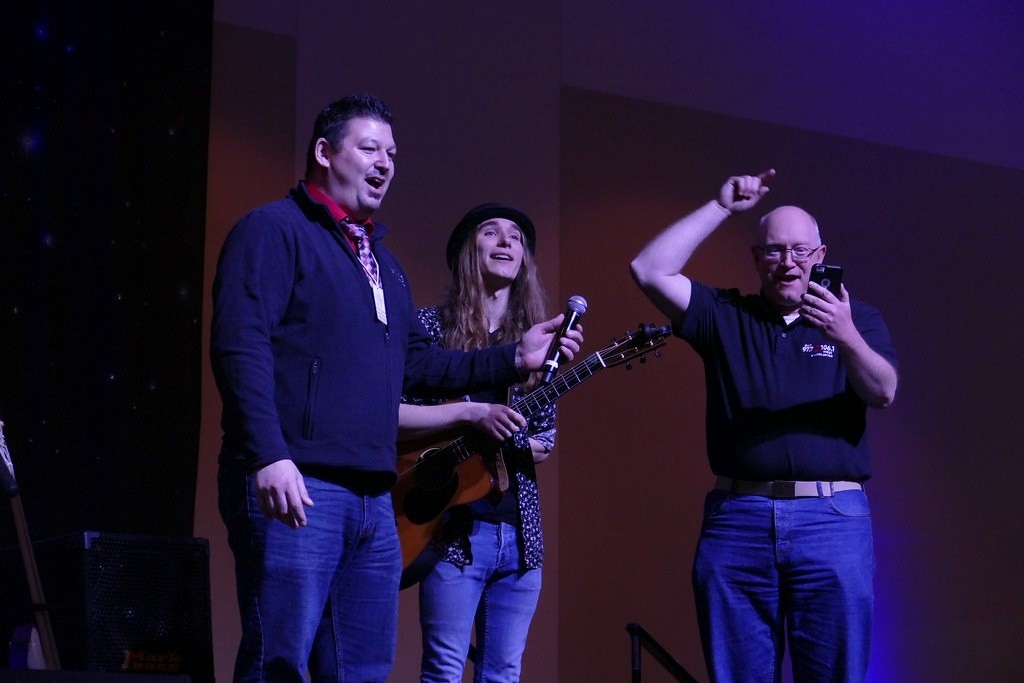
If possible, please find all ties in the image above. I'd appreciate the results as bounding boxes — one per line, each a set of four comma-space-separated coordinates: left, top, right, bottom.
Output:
341, 219, 382, 294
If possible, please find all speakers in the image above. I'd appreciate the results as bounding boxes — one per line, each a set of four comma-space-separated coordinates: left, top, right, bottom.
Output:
0, 529, 216, 683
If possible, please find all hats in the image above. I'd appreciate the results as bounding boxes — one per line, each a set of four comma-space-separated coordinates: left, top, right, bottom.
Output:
446, 203, 538, 279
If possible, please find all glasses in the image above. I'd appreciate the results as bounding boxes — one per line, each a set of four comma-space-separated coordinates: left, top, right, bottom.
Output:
757, 245, 819, 262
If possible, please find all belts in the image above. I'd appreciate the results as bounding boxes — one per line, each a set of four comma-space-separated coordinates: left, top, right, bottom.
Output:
717, 477, 864, 501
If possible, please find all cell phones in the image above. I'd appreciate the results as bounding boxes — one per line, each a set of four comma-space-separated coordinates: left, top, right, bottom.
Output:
802, 263, 843, 329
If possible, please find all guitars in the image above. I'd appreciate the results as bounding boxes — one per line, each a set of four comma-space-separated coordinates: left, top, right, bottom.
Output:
388, 323, 673, 592
1, 422, 65, 672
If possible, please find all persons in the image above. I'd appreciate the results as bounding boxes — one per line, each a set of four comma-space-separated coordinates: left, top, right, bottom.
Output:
210, 94, 584, 682
630, 169, 898, 682
394, 203, 558, 681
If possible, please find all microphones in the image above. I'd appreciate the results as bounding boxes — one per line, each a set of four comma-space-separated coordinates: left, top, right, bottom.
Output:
541, 295, 587, 384
